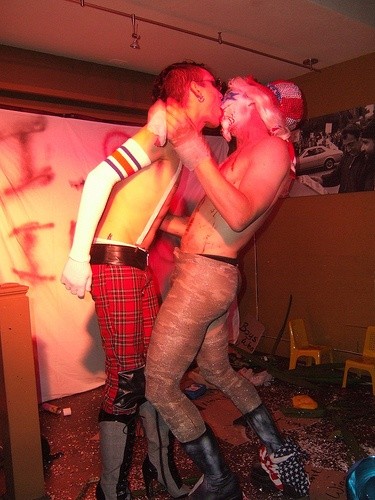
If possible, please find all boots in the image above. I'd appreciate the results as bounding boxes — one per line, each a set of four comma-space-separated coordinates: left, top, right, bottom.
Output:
179, 424, 241, 500
139, 399, 190, 499
235, 402, 312, 497
94, 413, 133, 500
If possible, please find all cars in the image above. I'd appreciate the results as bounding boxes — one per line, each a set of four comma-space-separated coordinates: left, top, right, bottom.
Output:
298, 145, 344, 173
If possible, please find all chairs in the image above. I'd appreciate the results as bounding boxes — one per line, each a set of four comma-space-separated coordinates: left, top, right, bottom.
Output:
288, 320, 335, 371
342, 326, 375, 396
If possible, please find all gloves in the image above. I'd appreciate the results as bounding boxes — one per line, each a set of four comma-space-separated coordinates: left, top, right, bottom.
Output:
60, 258, 93, 297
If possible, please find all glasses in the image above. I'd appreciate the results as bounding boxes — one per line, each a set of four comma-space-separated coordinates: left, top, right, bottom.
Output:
200, 77, 223, 91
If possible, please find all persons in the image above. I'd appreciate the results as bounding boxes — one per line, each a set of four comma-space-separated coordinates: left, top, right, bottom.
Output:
294, 119, 375, 194
143, 76, 311, 500
60, 61, 226, 500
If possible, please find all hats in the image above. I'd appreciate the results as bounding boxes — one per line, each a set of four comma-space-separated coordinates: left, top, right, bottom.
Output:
269, 80, 303, 131
